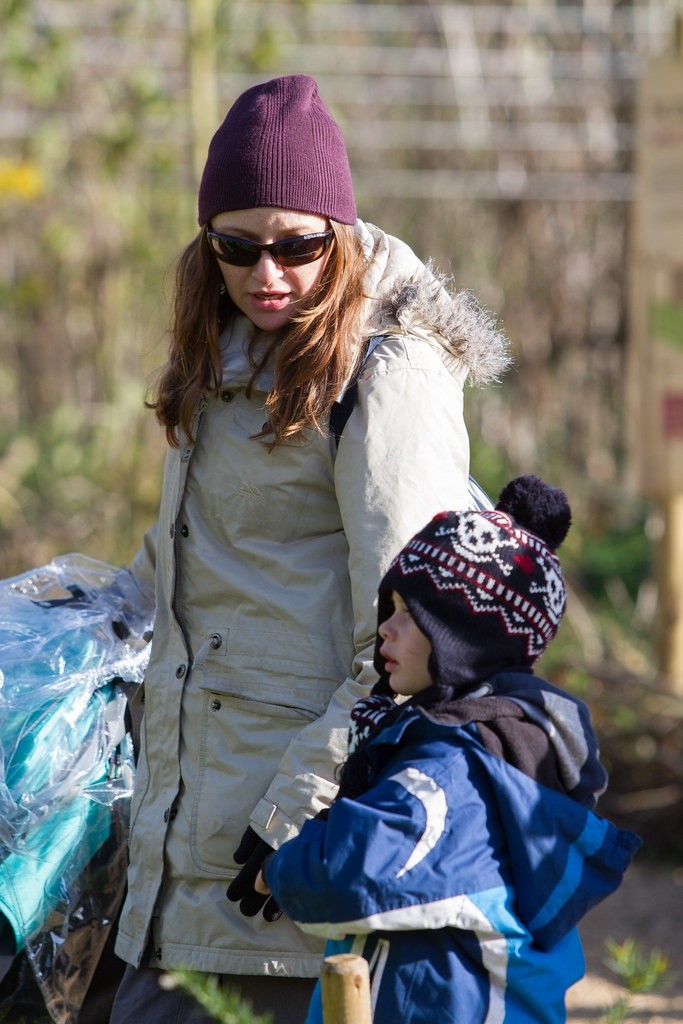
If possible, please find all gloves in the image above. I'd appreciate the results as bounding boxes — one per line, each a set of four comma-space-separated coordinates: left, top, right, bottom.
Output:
226, 825, 283, 921
30, 583, 133, 640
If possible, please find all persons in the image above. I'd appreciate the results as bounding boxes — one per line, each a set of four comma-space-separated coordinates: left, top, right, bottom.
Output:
91, 76, 513, 1024
255, 477, 642, 1023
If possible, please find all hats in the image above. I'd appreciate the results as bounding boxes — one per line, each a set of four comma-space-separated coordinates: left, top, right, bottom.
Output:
197, 74, 357, 225
333, 474, 572, 802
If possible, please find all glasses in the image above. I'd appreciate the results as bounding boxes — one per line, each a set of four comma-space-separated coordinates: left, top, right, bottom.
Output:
205, 223, 338, 268
